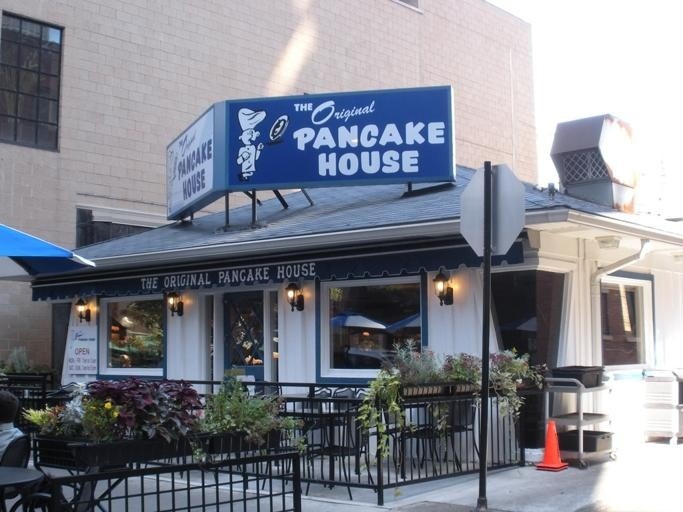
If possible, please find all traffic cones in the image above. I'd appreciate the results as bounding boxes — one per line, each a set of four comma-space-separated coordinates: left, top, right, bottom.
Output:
535, 420, 569, 472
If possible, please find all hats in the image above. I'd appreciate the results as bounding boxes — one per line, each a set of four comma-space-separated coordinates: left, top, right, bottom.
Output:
0, 390, 19, 422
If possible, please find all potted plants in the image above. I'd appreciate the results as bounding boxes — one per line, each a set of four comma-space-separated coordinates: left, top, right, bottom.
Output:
22, 377, 307, 471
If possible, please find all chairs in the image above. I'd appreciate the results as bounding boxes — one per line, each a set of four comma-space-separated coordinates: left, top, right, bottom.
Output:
246, 381, 479, 499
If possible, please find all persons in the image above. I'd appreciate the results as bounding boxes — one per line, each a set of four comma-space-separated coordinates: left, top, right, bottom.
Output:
0, 390, 24, 458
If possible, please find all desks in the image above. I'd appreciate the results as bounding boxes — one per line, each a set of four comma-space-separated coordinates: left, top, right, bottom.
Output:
0, 465, 42, 491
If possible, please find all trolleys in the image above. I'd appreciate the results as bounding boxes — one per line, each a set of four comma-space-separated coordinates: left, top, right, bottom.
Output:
543, 376, 617, 469
641, 368, 683, 445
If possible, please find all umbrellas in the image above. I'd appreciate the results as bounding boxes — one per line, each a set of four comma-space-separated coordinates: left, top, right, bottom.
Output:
516, 316, 536, 335
328, 310, 389, 333
0, 223, 96, 284
383, 308, 421, 332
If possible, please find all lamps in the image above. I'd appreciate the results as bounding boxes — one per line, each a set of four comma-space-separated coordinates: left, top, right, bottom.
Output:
286, 283, 304, 312
432, 271, 453, 308
165, 293, 183, 316
75, 298, 88, 323
